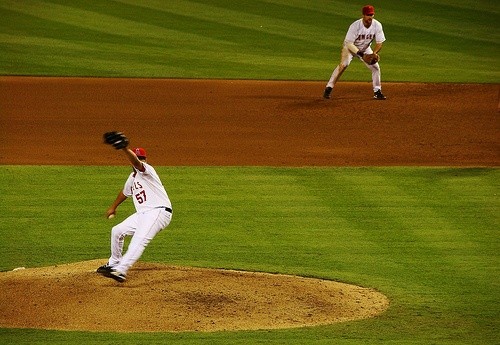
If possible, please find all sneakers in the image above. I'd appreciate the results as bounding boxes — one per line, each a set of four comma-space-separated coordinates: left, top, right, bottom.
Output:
374, 89, 386, 99
97, 263, 116, 273
104, 270, 126, 282
324, 87, 332, 99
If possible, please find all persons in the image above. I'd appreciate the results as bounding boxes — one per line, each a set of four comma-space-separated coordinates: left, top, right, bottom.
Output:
323, 6, 387, 100
96, 130, 173, 283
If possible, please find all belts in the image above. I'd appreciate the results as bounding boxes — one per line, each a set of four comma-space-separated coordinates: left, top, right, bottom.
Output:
157, 207, 172, 213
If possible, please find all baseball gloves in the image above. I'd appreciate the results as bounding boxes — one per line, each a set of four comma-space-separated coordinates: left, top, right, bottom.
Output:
363, 54, 379, 65
103, 131, 129, 150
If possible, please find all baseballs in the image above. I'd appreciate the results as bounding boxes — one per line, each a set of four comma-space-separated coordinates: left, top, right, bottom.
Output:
108, 214, 115, 219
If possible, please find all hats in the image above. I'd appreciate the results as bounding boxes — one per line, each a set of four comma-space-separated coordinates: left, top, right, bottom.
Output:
132, 148, 146, 158
362, 5, 375, 15
